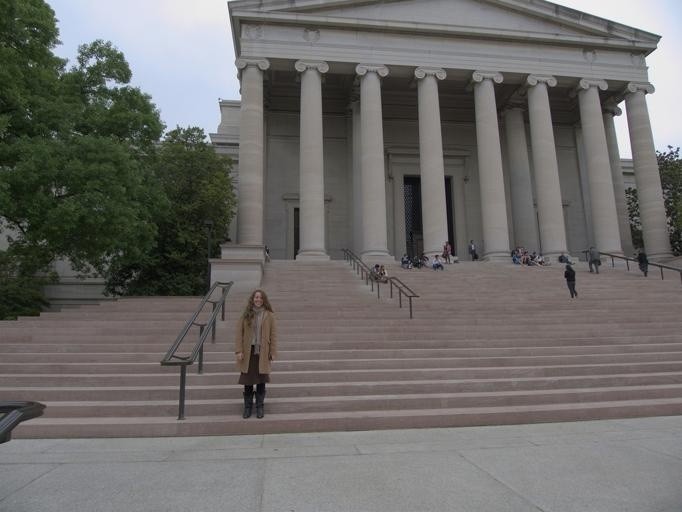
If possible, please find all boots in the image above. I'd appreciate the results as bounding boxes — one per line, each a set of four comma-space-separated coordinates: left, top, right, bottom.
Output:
254, 392, 265, 418
243, 391, 255, 418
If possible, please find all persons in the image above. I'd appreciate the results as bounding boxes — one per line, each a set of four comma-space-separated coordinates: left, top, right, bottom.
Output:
634, 249, 649, 278
369, 237, 548, 283
558, 251, 576, 265
587, 246, 601, 274
563, 264, 580, 299
233, 288, 277, 420
265, 245, 272, 258
